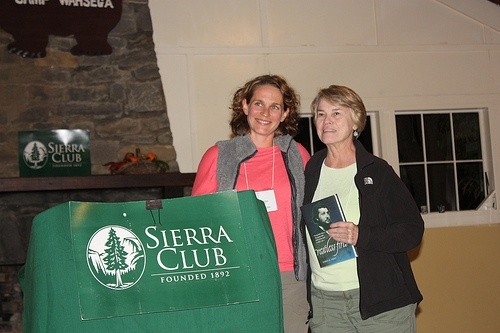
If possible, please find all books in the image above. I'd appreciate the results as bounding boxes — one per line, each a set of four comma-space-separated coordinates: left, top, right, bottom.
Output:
300, 194, 359, 268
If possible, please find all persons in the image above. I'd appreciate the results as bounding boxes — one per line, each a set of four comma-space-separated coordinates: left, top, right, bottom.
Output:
191, 74, 310, 333
303, 85, 423, 333
